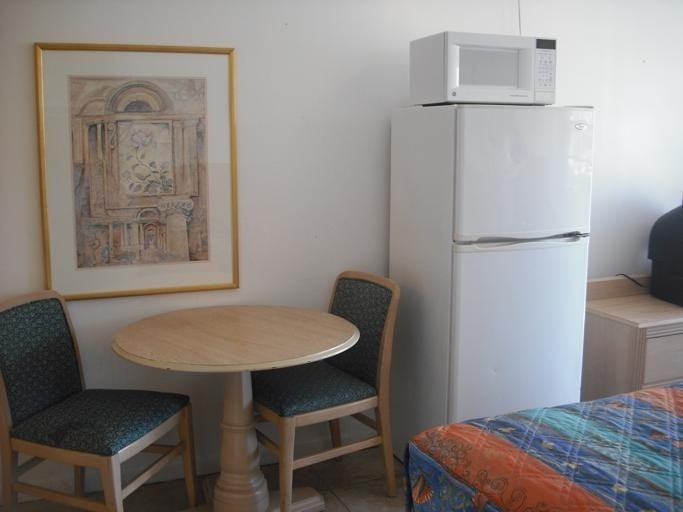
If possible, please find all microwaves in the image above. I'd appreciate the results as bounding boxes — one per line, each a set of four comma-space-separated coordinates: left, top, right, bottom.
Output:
408, 30, 558, 105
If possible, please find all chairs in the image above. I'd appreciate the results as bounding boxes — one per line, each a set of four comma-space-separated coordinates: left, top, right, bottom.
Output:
0, 289, 197, 512
252, 271, 404, 511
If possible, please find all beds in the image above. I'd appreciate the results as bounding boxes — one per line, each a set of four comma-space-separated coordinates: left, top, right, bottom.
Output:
395, 386, 682, 512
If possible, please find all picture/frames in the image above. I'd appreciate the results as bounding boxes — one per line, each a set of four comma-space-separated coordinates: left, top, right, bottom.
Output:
33, 40, 237, 301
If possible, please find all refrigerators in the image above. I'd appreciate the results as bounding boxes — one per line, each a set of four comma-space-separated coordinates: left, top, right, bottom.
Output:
387, 103, 594, 466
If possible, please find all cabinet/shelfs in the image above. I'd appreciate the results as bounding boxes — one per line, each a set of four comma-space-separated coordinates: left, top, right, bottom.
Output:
580, 275, 682, 405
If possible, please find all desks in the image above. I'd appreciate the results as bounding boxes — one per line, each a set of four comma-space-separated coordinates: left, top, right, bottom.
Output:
109, 303, 360, 512
